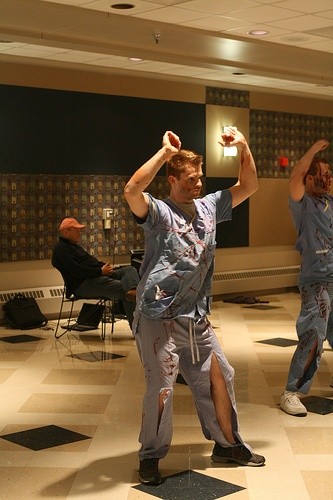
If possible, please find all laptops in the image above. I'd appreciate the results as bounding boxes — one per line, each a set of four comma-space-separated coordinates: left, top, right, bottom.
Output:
61, 303, 105, 331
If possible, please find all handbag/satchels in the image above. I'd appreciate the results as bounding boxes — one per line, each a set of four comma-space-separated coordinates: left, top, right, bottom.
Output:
3, 293, 48, 330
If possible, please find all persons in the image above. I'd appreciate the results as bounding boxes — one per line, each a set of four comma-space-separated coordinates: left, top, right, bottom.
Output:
51, 217, 141, 339
124, 123, 267, 483
278, 138, 333, 416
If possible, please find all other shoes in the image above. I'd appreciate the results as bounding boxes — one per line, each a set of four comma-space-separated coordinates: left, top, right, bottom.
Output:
126, 290, 136, 303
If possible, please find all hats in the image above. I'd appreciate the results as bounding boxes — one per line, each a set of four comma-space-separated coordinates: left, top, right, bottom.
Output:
59, 218, 86, 231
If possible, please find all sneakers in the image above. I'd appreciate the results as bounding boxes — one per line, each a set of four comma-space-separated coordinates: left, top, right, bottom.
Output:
138, 458, 162, 484
280, 387, 307, 416
211, 442, 265, 466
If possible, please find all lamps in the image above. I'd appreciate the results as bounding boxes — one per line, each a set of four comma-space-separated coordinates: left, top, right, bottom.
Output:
223, 125, 238, 156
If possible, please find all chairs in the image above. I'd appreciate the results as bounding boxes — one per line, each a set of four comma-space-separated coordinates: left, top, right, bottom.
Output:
56, 281, 115, 341
